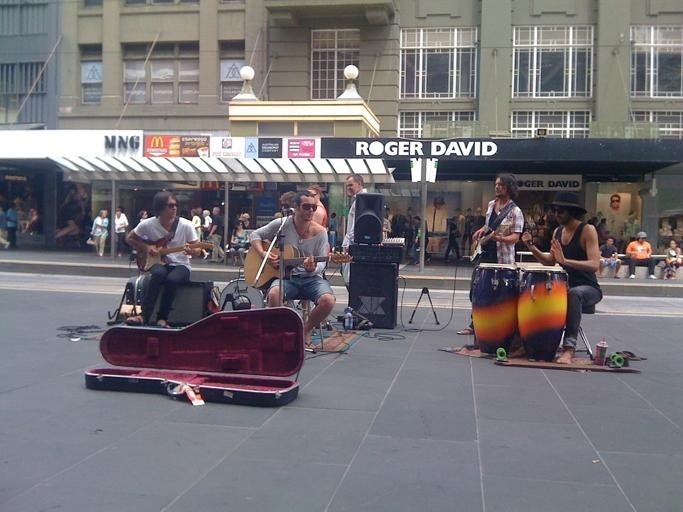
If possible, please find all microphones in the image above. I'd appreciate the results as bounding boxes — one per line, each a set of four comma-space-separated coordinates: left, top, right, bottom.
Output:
276, 208, 296, 235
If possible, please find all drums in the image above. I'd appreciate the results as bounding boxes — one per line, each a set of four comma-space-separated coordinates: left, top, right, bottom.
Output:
237, 227, 255, 248
469, 262, 519, 354
519, 264, 569, 361
218, 278, 264, 312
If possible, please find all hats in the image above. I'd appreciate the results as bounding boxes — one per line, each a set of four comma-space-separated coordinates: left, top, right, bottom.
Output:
543, 190, 587, 216
637, 231, 647, 239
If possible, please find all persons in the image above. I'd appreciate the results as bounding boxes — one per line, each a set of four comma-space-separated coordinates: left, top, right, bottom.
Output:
463, 206, 475, 261
247, 189, 337, 352
124, 190, 201, 327
307, 184, 327, 230
626, 232, 657, 279
7, 203, 18, 246
202, 210, 214, 263
454, 208, 466, 260
280, 192, 297, 214
209, 207, 227, 264
91, 209, 108, 256
392, 207, 405, 239
340, 174, 369, 320
405, 207, 415, 256
606, 195, 628, 235
444, 217, 462, 262
138, 208, 149, 222
474, 207, 486, 233
521, 189, 603, 364
230, 221, 250, 267
382, 215, 391, 238
596, 217, 608, 242
56, 185, 89, 235
598, 237, 620, 279
665, 240, 682, 279
0, 205, 11, 249
326, 211, 337, 254
459, 172, 524, 335
241, 212, 253, 229
115, 206, 130, 257
414, 216, 432, 266
190, 209, 202, 244
196, 208, 206, 224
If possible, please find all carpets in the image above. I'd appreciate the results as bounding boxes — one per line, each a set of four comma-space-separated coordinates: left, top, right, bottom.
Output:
440, 340, 640, 378
307, 323, 371, 354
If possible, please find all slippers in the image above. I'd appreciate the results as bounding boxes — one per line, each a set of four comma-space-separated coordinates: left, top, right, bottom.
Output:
158, 320, 170, 329
457, 327, 474, 335
124, 314, 143, 325
303, 339, 316, 349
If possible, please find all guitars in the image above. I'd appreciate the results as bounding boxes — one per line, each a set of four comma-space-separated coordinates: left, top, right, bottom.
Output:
242, 237, 354, 291
469, 226, 502, 260
131, 237, 214, 272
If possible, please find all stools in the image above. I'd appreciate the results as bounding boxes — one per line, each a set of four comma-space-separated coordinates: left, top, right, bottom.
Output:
289, 299, 323, 348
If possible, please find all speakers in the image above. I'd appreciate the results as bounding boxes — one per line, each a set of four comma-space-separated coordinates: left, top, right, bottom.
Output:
354, 193, 385, 245
349, 262, 399, 329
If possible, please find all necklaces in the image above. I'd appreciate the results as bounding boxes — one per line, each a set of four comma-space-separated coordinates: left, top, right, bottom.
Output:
495, 199, 512, 214
563, 225, 581, 249
292, 219, 311, 245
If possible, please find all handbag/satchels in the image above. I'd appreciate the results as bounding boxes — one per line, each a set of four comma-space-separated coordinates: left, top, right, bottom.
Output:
86, 237, 97, 245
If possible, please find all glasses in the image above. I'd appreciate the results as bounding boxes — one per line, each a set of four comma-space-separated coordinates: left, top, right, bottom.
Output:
164, 203, 180, 209
302, 202, 318, 211
550, 206, 563, 215
280, 203, 289, 209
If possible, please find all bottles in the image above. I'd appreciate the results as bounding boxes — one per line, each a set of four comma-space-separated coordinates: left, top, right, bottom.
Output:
345, 307, 353, 331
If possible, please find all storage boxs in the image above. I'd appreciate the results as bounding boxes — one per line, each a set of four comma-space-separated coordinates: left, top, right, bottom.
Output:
147, 276, 214, 328
347, 260, 399, 329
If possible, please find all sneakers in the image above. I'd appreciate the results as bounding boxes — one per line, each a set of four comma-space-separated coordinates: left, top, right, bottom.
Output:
118, 254, 122, 257
96, 251, 99, 255
628, 274, 635, 279
204, 253, 210, 259
647, 275, 656, 280
556, 347, 575, 364
99, 252, 104, 256
